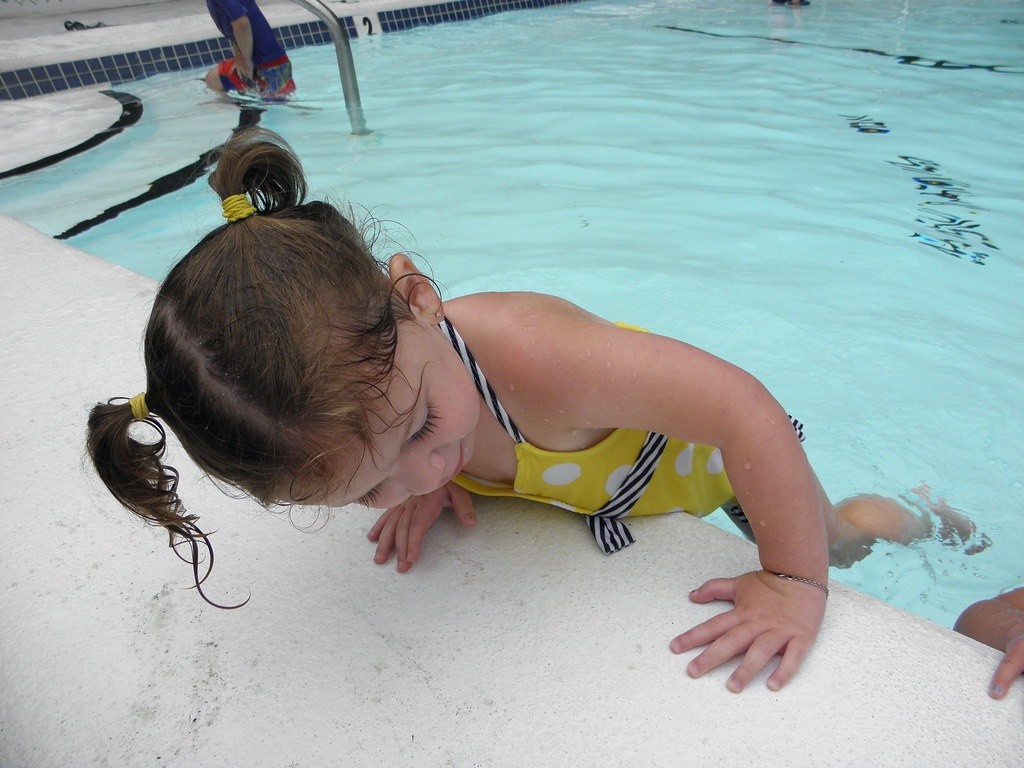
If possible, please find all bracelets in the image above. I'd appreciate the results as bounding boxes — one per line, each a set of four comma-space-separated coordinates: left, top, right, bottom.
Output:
774, 572, 829, 599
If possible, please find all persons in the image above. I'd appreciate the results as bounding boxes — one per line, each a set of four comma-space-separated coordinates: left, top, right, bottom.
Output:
206, 0, 295, 100
85, 127, 915, 693
